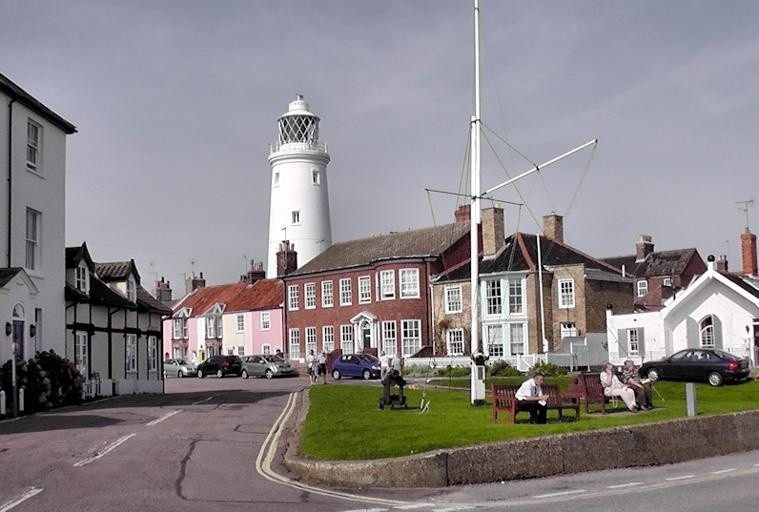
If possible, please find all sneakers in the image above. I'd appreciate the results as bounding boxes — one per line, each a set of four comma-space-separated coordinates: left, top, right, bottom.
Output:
629, 404, 652, 412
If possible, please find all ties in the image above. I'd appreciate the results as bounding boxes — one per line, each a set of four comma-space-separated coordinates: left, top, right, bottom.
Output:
534, 386, 537, 396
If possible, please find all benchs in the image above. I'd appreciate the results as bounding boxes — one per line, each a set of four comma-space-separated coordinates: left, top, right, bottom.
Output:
581, 371, 652, 415
490, 382, 580, 425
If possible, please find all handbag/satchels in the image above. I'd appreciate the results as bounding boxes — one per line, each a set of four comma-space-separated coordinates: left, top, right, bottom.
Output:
306, 368, 312, 375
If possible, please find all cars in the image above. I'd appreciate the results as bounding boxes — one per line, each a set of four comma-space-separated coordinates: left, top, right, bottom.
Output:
331, 353, 381, 381
640, 346, 750, 385
163, 352, 297, 381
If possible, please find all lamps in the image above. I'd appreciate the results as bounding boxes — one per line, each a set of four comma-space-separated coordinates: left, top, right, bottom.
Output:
6, 322, 11, 336
30, 324, 36, 337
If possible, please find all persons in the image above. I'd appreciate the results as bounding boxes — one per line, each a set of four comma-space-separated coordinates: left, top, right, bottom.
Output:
515, 373, 549, 424
317, 353, 327, 384
600, 363, 639, 413
307, 350, 317, 386
621, 360, 652, 411
